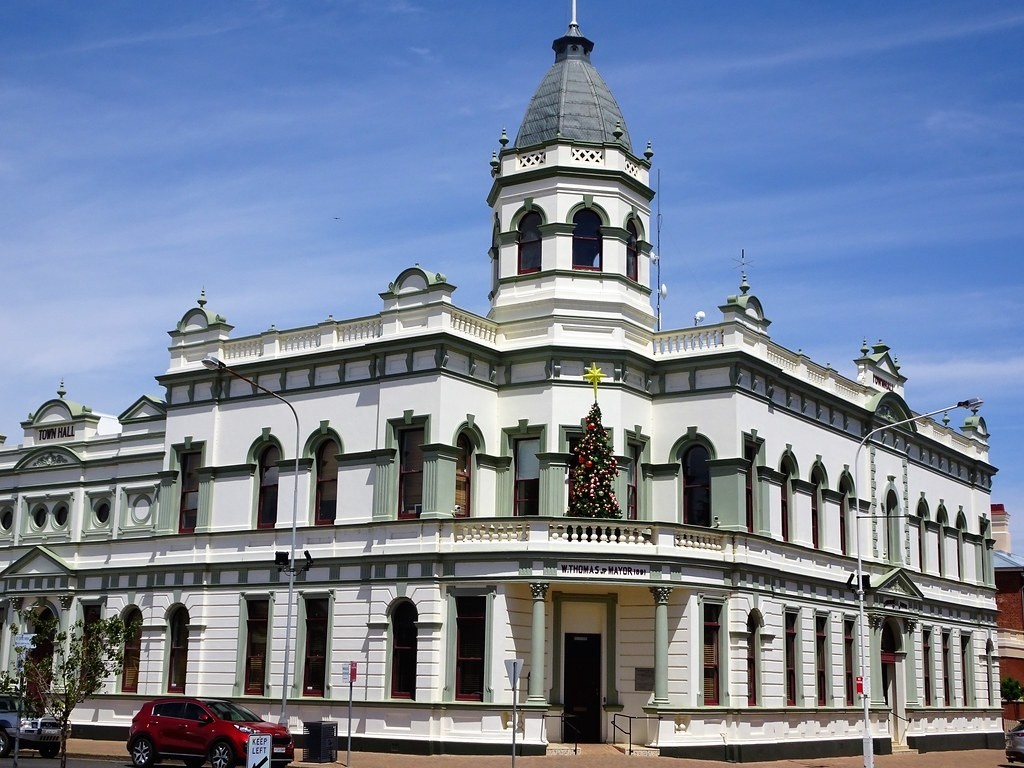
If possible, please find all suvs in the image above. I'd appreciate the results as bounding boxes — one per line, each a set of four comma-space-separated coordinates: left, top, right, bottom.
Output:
0, 690, 74, 758
126, 696, 295, 768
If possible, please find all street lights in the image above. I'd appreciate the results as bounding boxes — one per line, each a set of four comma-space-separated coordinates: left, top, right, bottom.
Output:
852, 395, 985, 767
200, 354, 302, 726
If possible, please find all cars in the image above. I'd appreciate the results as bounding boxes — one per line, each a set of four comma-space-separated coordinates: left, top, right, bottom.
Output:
1004, 719, 1024, 763
